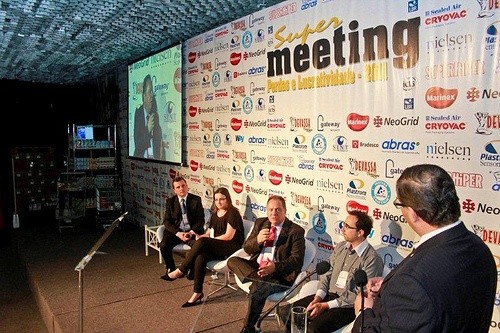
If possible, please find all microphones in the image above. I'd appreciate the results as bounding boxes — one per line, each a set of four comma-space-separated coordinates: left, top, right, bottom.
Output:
184, 232, 193, 239
263, 222, 270, 246
256, 260, 330, 333
354, 269, 368, 333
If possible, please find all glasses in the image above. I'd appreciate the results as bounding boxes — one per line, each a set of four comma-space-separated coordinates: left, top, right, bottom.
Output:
393, 198, 413, 208
343, 222, 357, 230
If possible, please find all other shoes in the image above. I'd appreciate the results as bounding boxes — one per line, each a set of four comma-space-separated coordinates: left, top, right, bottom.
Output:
240, 326, 255, 333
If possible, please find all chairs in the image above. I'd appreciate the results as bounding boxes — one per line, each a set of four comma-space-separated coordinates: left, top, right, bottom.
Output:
145, 209, 390, 333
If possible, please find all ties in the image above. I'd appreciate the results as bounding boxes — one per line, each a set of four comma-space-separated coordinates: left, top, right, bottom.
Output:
260, 226, 276, 267
181, 199, 187, 214
146, 115, 151, 149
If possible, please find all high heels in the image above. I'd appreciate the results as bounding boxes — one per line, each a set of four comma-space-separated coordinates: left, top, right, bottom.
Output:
160, 272, 186, 281
181, 291, 204, 307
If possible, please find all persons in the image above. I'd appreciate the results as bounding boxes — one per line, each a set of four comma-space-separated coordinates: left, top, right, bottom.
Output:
343, 164, 497, 333
160, 177, 205, 280
227, 196, 306, 333
134, 75, 162, 159
280, 211, 383, 333
160, 188, 244, 307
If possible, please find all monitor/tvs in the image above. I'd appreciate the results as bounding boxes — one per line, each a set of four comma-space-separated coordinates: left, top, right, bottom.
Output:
76, 125, 94, 141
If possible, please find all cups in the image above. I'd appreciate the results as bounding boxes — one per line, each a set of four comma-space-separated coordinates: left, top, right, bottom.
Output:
290, 306, 307, 333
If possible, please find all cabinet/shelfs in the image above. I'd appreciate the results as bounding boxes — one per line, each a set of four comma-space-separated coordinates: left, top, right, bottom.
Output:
56, 123, 122, 232
